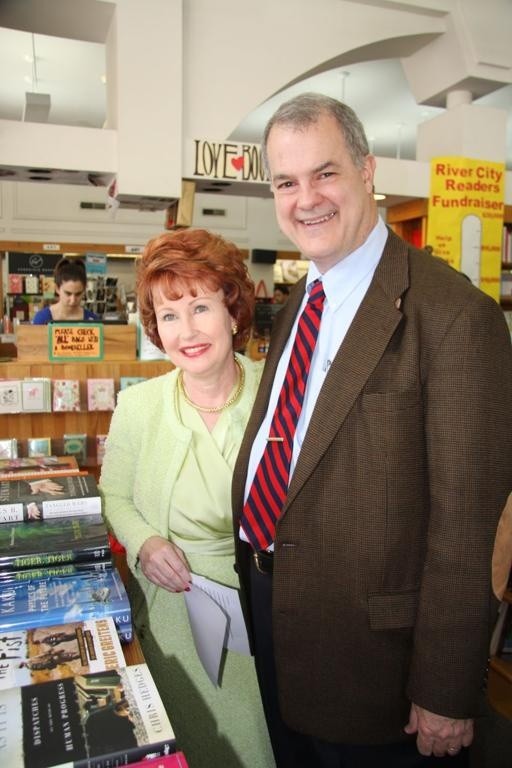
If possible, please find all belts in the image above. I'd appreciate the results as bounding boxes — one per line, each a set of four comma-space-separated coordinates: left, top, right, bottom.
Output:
251, 549, 275, 577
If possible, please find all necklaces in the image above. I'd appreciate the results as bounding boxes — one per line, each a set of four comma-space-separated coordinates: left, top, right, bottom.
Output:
179, 355, 245, 412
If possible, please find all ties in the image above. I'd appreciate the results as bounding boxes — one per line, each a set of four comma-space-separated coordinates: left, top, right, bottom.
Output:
237, 280, 326, 553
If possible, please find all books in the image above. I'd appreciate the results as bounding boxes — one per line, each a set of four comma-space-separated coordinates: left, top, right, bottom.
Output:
502, 226, 511, 335
8, 274, 118, 323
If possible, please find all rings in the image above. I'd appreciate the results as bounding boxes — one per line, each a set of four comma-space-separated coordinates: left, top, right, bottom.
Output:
449, 747, 461, 752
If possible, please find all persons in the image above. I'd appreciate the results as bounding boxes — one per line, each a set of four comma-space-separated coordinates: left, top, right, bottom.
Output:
32, 256, 100, 324
273, 285, 289, 305
233, 91, 512, 767
99, 227, 278, 768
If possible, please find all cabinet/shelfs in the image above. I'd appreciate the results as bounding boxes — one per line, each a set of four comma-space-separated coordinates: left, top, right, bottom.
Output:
385, 200, 511, 319
